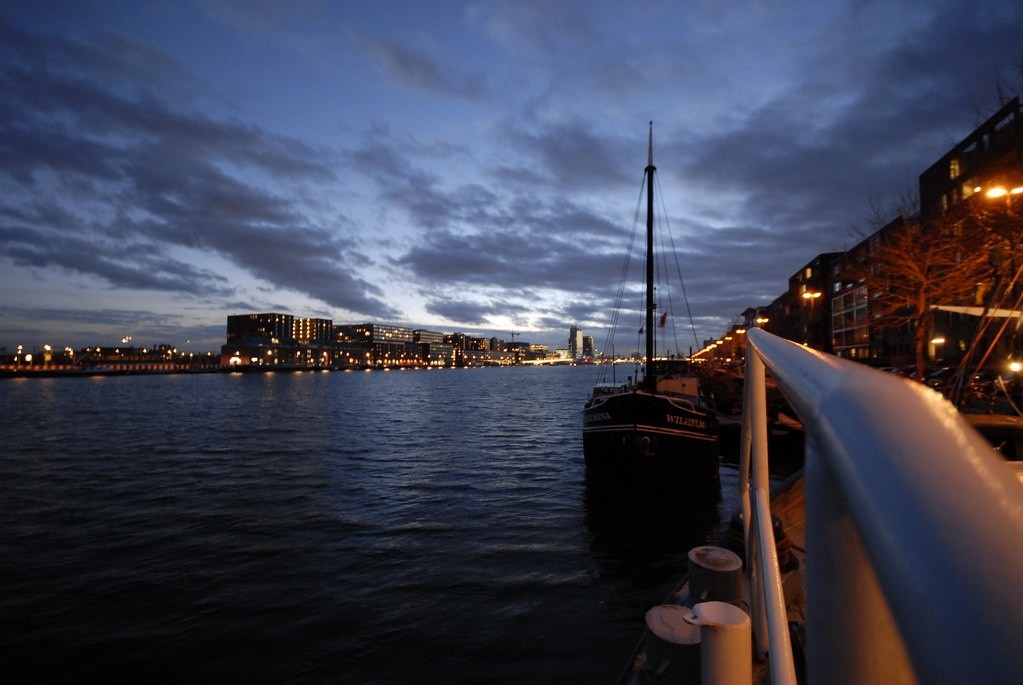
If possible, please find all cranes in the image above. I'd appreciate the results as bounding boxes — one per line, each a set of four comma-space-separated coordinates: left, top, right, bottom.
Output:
491, 329, 520, 343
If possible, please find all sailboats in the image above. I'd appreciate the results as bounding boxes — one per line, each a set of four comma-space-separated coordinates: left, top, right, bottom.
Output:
583, 120, 722, 456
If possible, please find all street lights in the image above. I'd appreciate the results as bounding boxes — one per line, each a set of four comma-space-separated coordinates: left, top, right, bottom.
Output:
17, 344, 23, 368
44, 344, 51, 370
802, 291, 822, 348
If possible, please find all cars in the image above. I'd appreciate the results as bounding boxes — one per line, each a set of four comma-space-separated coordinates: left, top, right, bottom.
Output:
876, 364, 1015, 399
